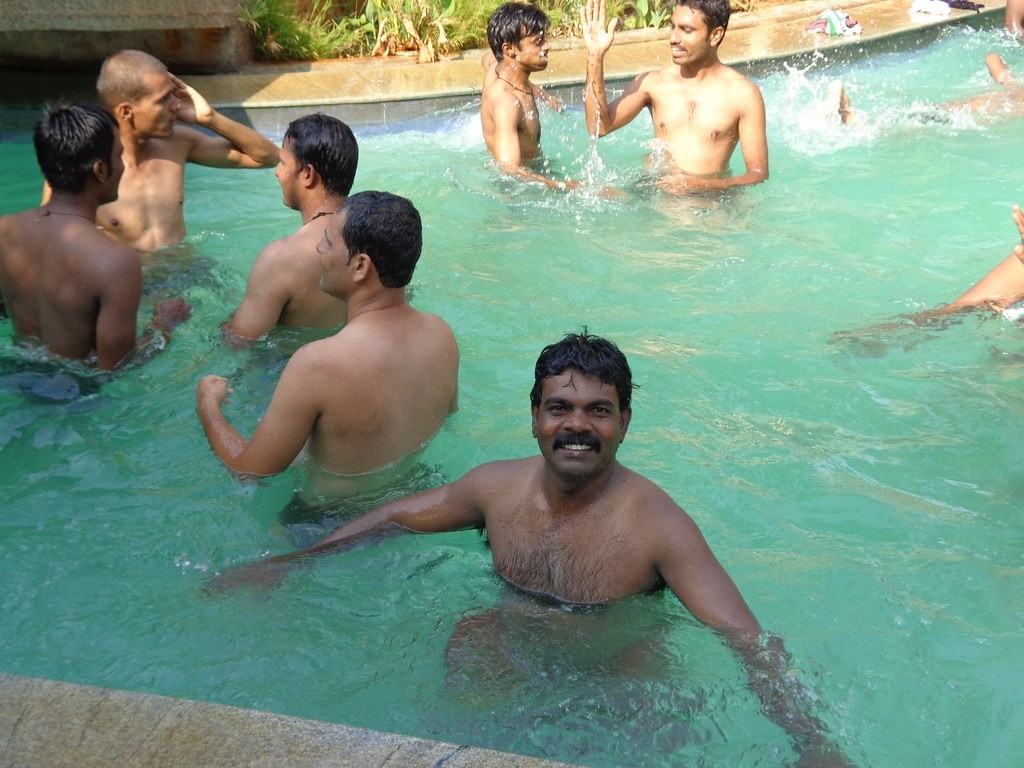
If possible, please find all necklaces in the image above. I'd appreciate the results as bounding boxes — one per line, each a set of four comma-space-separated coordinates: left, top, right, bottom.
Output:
495, 63, 533, 96
41, 206, 96, 223
302, 212, 334, 226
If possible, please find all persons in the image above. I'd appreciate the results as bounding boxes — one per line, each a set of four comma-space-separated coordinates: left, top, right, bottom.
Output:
1005, 0, 1024, 45
837, 53, 1024, 133
201, 324, 851, 768
579, 0, 769, 193
481, 0, 627, 199
828, 205, 1024, 344
41, 48, 283, 256
217, 111, 360, 347
194, 189, 459, 542
0, 99, 194, 412
